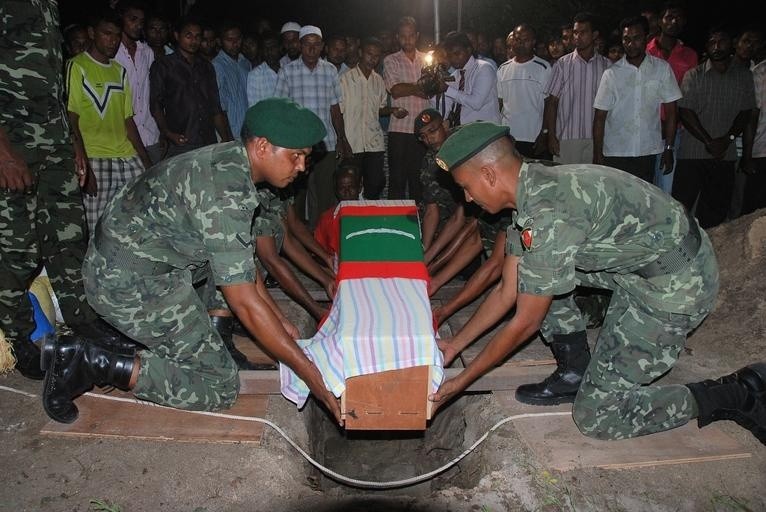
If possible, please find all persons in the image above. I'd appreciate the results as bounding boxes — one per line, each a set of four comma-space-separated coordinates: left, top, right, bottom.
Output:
1, 1, 765, 382
39, 98, 342, 427
429, 123, 766, 445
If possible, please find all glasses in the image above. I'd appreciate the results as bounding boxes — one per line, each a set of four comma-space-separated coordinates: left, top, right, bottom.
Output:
418, 126, 440, 141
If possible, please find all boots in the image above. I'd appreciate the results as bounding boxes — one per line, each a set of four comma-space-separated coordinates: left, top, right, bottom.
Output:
10, 337, 52, 379
210, 315, 276, 370
685, 362, 765, 446
515, 331, 592, 407
39, 332, 135, 424
99, 328, 139, 359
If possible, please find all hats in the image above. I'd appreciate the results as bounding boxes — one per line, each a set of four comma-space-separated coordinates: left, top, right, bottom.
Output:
414, 108, 440, 133
299, 25, 322, 40
435, 120, 510, 174
281, 22, 302, 33
246, 97, 327, 149
